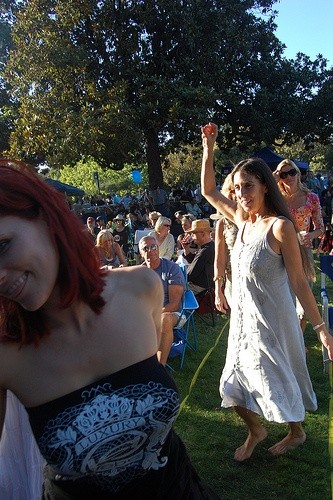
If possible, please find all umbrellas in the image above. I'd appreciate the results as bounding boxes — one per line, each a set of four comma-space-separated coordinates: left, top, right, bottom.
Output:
248, 146, 286, 171
41, 177, 86, 198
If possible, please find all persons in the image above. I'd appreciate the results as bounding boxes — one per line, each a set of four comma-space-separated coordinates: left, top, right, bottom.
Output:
137, 235, 186, 371
93, 229, 129, 271
270, 158, 326, 354
175, 213, 201, 270
294, 160, 333, 256
147, 217, 175, 261
180, 218, 216, 297
0, 156, 216, 500
69, 183, 217, 253
198, 122, 333, 462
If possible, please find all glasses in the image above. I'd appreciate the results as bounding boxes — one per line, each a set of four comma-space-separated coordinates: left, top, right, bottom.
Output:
141, 244, 160, 252
279, 169, 297, 179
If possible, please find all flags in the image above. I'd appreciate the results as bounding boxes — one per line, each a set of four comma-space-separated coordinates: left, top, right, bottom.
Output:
132, 169, 142, 185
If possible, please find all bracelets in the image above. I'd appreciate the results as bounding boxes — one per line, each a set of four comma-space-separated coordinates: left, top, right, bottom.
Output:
313, 322, 325, 330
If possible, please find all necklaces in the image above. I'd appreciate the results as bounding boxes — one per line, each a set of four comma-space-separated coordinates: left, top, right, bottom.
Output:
248, 210, 269, 236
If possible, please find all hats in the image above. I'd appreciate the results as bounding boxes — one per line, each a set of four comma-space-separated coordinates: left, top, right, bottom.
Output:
87, 217, 94, 222
185, 219, 213, 234
113, 214, 125, 221
96, 216, 104, 222
149, 211, 162, 220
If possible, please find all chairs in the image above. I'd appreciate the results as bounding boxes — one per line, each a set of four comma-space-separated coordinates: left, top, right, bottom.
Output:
164, 265, 199, 373
